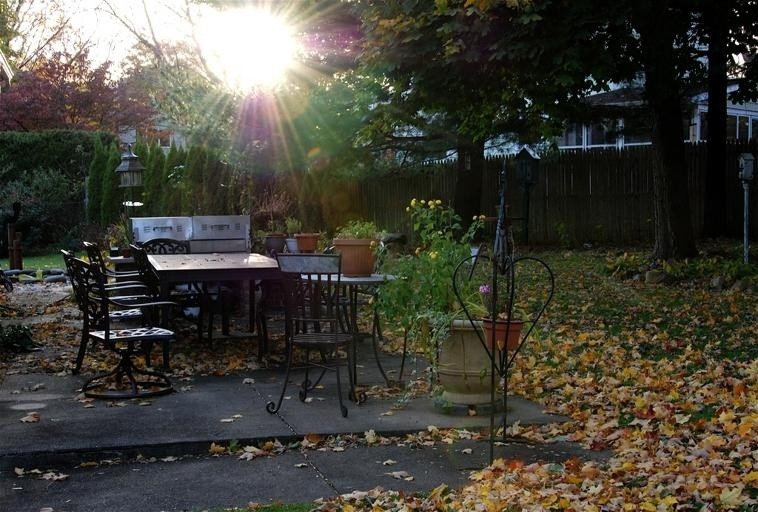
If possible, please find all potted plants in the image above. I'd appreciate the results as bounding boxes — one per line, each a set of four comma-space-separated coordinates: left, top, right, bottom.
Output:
261, 216, 321, 255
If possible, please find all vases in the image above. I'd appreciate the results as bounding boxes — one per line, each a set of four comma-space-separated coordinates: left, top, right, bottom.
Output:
481, 316, 524, 352
334, 239, 380, 277
435, 318, 506, 407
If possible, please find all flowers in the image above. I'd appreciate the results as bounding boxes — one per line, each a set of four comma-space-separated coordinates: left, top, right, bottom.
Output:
478, 282, 520, 319
367, 196, 487, 356
333, 218, 385, 253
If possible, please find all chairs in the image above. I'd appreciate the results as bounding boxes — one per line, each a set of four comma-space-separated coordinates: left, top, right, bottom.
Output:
255, 252, 366, 419
59, 237, 232, 401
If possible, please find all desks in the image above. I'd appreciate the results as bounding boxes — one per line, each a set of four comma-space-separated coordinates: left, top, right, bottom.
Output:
147, 252, 287, 374
298, 272, 398, 400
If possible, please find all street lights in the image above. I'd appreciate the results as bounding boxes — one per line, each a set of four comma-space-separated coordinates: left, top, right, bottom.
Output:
115, 143, 147, 217
